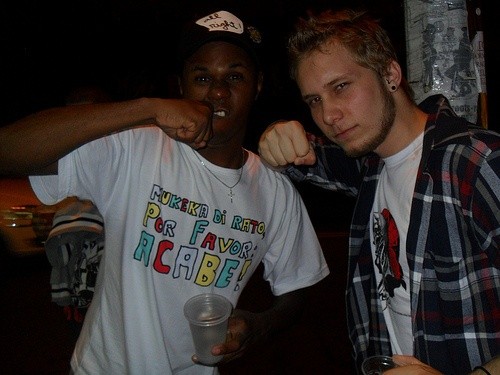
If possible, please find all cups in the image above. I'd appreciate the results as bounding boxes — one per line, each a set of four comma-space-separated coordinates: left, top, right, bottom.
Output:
361, 355, 400, 375
184, 294, 233, 366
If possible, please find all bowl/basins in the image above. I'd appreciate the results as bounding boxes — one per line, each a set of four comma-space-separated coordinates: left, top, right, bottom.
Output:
32, 212, 55, 238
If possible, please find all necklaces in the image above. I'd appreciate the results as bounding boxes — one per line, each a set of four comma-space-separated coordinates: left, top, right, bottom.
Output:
192, 143, 245, 202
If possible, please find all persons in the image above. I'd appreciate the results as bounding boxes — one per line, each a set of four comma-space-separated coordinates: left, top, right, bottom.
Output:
0, 12, 329, 375
258, 10, 500, 375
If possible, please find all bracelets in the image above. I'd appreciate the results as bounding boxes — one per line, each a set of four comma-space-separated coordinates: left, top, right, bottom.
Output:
471, 364, 490, 375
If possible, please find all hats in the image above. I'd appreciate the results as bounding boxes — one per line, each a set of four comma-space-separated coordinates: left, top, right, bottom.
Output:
171, 8, 266, 73
